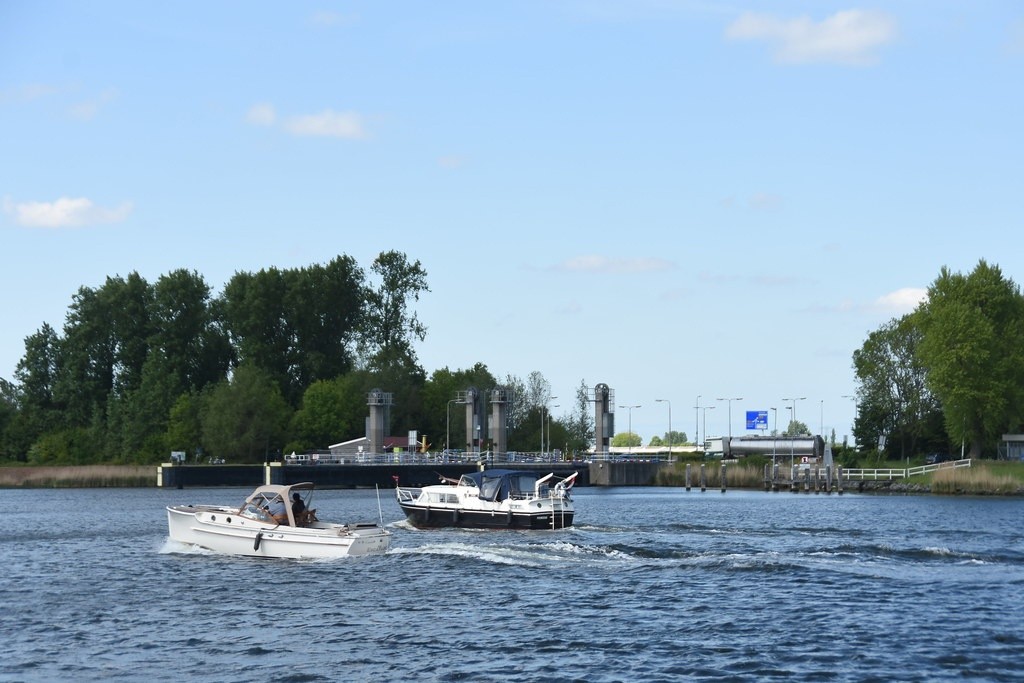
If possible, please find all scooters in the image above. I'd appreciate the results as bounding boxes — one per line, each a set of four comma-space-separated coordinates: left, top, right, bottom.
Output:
209, 455, 226, 465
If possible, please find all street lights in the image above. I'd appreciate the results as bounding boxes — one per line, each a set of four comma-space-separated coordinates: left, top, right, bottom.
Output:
782, 396, 807, 480
696, 394, 702, 457
786, 407, 794, 469
820, 400, 824, 438
716, 396, 743, 442
547, 404, 560, 459
541, 395, 557, 461
840, 393, 870, 430
619, 405, 642, 455
770, 407, 776, 479
694, 405, 716, 453
655, 399, 672, 461
447, 398, 462, 463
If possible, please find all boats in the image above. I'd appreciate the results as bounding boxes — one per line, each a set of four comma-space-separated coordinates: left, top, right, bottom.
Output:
393, 468, 580, 532
166, 463, 391, 563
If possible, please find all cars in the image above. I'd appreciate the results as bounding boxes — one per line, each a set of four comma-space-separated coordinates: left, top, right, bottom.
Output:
926, 452, 960, 467
440, 449, 596, 466
615, 451, 638, 462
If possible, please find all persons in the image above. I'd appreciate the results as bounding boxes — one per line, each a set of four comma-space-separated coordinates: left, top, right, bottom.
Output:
266, 494, 288, 525
291, 493, 305, 517
